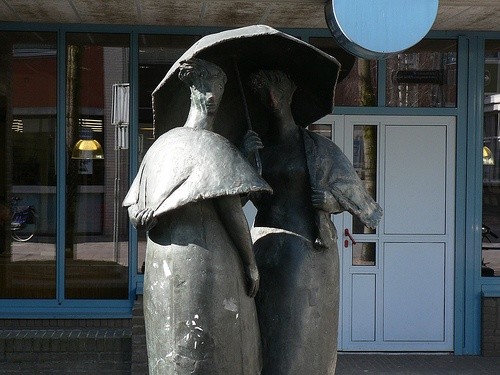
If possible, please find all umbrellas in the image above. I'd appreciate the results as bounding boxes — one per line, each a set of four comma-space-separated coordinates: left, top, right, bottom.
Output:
150, 25, 341, 175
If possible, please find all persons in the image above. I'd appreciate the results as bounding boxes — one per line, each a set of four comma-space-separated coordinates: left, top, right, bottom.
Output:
236, 61, 384, 375
121, 60, 273, 375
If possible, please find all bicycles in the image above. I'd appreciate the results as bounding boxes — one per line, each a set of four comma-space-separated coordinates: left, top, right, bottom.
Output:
10, 196, 41, 243
